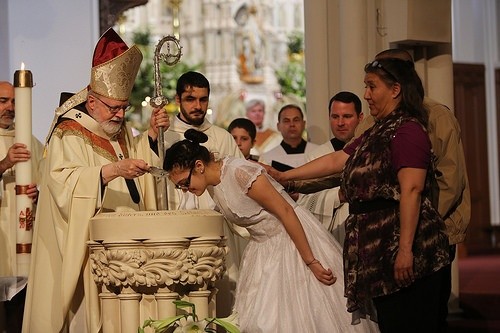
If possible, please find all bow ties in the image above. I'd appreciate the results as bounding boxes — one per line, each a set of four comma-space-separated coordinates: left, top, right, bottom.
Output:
109, 132, 121, 141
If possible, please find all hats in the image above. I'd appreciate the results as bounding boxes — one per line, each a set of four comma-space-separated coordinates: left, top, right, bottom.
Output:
90, 27, 143, 101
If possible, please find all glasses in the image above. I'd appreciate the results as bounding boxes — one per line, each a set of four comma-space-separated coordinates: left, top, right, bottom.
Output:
175, 165, 194, 190
365, 61, 399, 83
90, 94, 133, 113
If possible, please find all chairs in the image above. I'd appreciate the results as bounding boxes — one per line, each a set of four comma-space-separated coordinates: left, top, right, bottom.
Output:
447, 224, 500, 319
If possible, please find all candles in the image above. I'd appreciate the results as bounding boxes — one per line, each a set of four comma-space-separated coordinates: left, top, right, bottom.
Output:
10, 62, 34, 284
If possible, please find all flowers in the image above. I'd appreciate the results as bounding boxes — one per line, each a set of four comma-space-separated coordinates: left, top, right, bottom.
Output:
138, 300, 240, 333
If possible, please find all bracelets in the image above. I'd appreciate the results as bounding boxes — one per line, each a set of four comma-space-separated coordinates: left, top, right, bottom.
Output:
306, 258, 321, 266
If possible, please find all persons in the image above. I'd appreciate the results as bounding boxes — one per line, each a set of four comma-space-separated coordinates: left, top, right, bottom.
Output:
227, 118, 259, 162
245, 99, 282, 155
0, 81, 45, 332
164, 72, 245, 210
22, 27, 171, 333
247, 57, 451, 333
295, 91, 364, 247
257, 105, 318, 202
162, 129, 380, 333
277, 49, 471, 262
